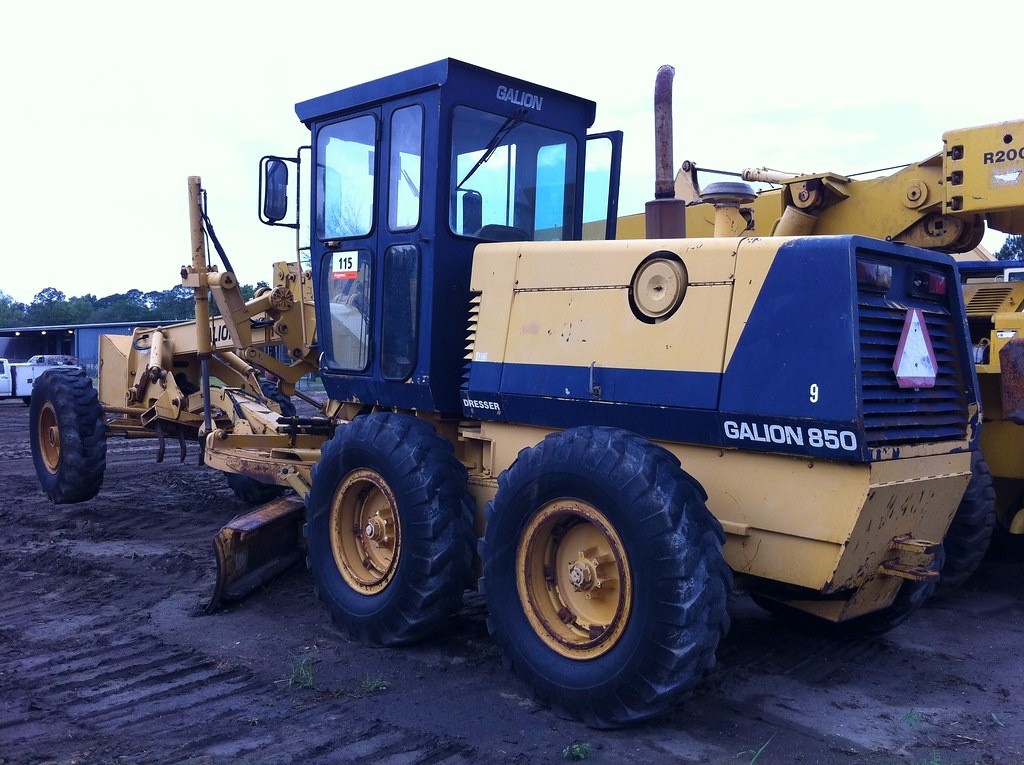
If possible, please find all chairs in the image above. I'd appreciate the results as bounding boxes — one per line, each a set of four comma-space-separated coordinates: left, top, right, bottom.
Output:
473, 224, 531, 241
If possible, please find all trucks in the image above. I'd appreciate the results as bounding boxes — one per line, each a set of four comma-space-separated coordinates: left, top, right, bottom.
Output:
0, 358, 80, 399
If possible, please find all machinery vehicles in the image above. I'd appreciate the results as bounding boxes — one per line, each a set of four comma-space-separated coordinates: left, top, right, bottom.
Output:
28, 56, 998, 732
534, 122, 1023, 604
